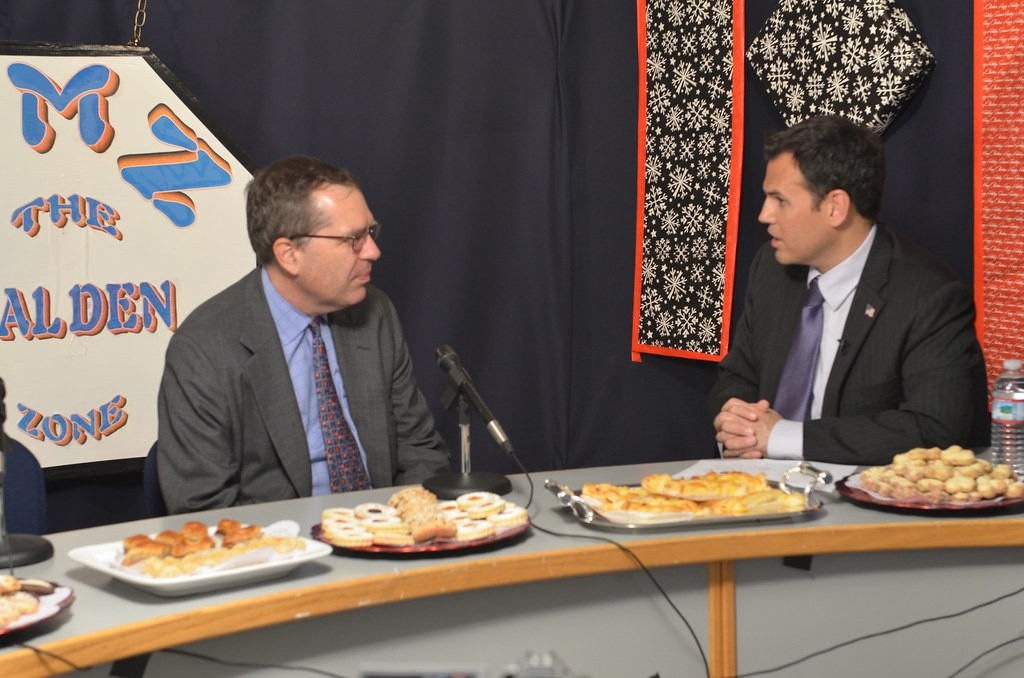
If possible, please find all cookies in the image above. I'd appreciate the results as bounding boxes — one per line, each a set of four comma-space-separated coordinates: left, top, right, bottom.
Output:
0, 575, 56, 626
318, 485, 528, 548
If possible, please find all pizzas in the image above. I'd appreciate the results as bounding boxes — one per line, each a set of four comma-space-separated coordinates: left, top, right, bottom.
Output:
578, 469, 807, 520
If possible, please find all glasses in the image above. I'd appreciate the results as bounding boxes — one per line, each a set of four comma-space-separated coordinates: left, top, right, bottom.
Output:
284, 229, 376, 256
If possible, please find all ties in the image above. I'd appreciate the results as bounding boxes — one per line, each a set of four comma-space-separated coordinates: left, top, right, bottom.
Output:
305, 315, 371, 496
771, 276, 823, 429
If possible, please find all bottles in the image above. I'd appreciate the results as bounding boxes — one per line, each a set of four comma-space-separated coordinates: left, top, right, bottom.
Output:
990, 359, 1024, 474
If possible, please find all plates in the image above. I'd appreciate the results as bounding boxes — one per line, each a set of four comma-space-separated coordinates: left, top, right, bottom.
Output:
66, 523, 333, 597
836, 472, 1024, 510
310, 499, 530, 552
0, 578, 75, 635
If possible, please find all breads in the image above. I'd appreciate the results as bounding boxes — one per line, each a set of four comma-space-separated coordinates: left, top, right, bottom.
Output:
119, 519, 305, 577
860, 445, 1024, 503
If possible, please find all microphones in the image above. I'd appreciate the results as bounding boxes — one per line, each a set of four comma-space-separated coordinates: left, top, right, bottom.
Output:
0, 377, 7, 476
434, 345, 514, 454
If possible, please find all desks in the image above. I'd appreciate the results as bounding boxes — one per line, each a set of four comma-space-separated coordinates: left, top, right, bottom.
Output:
0, 456, 1024, 678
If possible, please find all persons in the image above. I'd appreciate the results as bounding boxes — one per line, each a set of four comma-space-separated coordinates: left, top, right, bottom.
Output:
156, 158, 448, 516
710, 116, 991, 466
